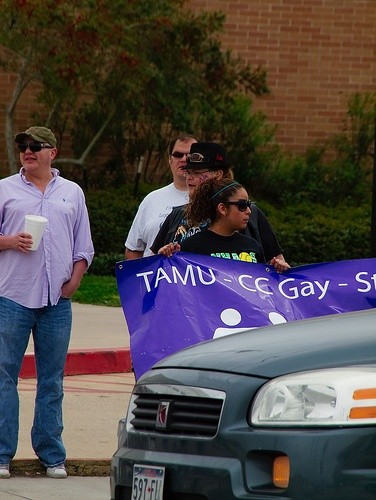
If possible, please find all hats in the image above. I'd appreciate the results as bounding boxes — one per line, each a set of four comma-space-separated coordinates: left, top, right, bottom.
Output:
15, 126, 57, 148
180, 142, 230, 169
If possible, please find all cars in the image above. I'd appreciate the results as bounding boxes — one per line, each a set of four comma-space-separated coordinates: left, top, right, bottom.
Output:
107, 307, 376, 500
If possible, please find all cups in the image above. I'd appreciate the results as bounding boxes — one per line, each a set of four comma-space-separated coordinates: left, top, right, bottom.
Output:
24, 214, 48, 251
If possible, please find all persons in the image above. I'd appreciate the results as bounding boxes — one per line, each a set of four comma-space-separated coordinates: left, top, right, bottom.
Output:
149, 143, 286, 263
0, 126, 94, 478
124, 135, 198, 372
157, 177, 292, 274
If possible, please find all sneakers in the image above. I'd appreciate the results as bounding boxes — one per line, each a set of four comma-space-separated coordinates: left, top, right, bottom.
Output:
46, 463, 67, 478
0, 464, 10, 478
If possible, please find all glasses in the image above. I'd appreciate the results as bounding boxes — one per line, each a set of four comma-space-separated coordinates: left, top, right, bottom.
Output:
18, 143, 53, 152
171, 152, 190, 158
183, 171, 207, 177
219, 199, 251, 212
